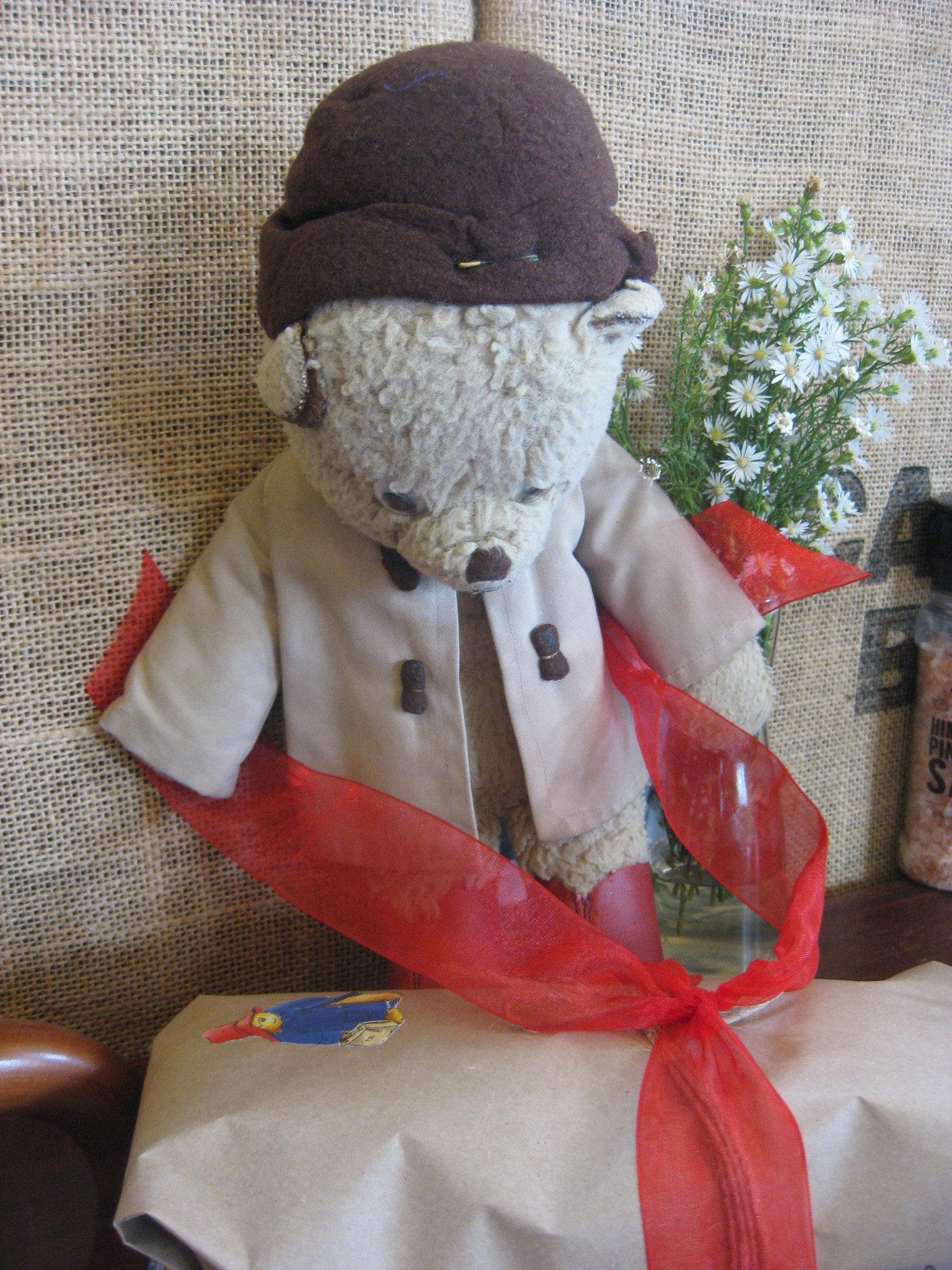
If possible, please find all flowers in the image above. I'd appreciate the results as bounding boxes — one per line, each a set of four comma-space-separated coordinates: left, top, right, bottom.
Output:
612, 173, 950, 939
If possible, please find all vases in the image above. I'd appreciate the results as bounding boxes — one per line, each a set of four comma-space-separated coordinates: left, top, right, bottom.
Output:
644, 601, 789, 1024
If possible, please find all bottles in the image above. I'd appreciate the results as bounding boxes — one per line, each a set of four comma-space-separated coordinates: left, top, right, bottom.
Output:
895, 586, 952, 892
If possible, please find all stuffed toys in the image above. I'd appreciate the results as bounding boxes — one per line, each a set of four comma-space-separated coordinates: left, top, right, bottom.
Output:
96, 46, 775, 966
